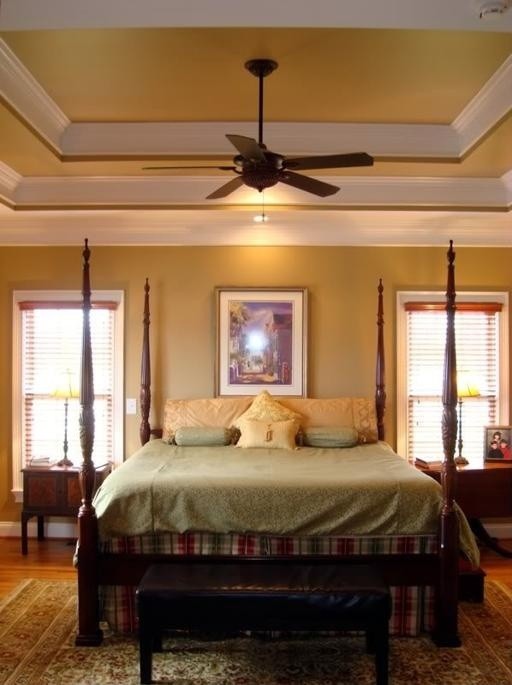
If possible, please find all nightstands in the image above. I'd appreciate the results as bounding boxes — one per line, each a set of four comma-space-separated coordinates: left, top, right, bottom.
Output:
20, 462, 111, 556
415, 462, 512, 526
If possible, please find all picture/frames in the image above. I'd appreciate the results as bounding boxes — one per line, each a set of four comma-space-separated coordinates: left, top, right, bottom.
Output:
214, 285, 309, 399
483, 425, 512, 463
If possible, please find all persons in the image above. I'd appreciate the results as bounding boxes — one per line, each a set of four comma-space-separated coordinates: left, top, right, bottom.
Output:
489, 432, 512, 458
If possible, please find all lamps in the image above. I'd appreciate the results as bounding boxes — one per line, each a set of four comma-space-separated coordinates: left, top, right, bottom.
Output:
456, 369, 480, 465
50, 368, 80, 467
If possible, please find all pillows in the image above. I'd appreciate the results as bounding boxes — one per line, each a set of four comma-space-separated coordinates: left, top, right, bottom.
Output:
162, 389, 379, 452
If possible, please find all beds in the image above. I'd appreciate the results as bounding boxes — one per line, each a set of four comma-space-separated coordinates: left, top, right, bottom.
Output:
73, 239, 487, 649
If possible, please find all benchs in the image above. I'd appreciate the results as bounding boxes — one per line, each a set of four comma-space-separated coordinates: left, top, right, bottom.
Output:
136, 563, 393, 685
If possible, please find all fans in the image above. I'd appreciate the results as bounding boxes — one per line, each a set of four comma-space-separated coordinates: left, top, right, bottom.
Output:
142, 59, 374, 199
